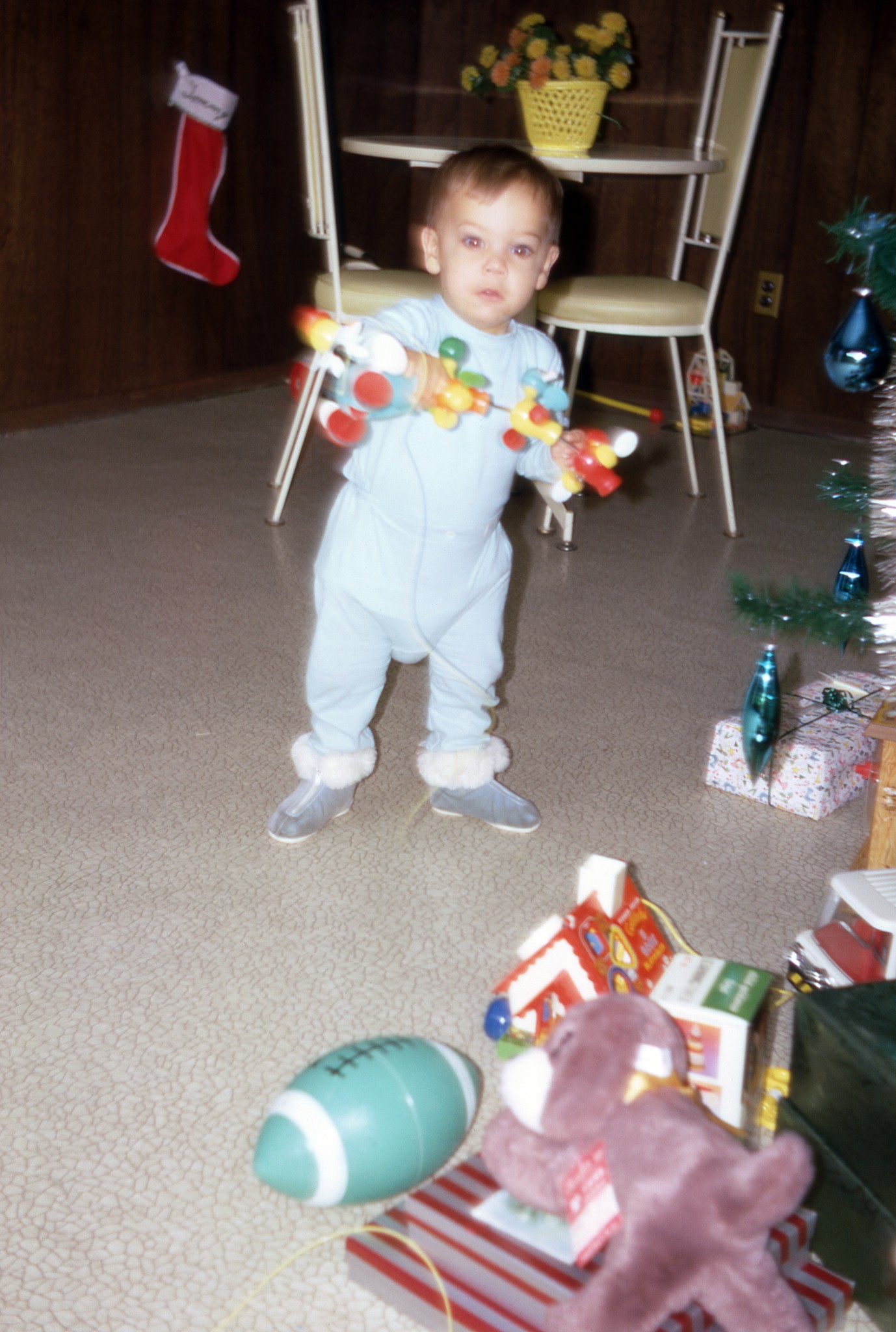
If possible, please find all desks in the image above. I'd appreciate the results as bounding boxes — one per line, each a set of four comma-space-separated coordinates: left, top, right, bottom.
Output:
341, 129, 725, 298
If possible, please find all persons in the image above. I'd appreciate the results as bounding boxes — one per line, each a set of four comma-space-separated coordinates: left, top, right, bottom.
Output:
269, 142, 583, 842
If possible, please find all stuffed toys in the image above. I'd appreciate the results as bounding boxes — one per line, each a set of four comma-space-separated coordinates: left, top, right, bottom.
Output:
482, 993, 819, 1332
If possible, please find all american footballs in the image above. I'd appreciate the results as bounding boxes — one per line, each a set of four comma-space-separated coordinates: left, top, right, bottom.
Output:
254, 1035, 484, 1206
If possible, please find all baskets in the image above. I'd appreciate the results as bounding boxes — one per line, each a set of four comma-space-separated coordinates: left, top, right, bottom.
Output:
515, 78, 609, 153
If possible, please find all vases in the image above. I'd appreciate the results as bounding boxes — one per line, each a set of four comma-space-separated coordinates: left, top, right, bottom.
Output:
513, 77, 611, 149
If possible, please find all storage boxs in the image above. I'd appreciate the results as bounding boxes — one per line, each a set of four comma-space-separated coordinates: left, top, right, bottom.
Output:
647, 945, 774, 1129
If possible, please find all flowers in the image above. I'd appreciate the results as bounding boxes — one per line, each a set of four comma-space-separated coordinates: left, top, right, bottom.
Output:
460, 6, 632, 102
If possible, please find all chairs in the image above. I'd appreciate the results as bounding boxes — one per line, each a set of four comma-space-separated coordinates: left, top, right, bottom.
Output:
275, 0, 388, 541
531, 1, 787, 553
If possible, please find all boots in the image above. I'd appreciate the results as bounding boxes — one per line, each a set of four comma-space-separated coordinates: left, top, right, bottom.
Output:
266, 733, 376, 842
415, 735, 544, 834
